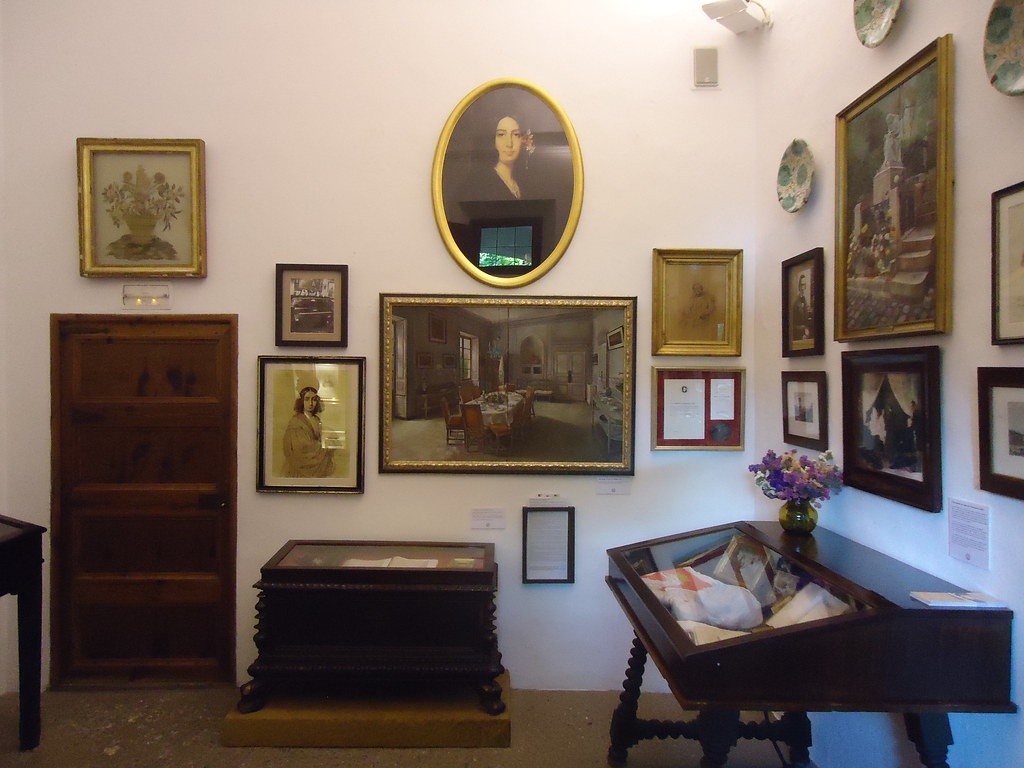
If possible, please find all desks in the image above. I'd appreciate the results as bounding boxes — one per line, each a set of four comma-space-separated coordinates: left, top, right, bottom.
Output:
238, 538, 507, 714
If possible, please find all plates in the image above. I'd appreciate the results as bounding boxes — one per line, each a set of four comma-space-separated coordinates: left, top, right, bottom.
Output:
852, 0, 901, 48
776, 136, 814, 214
982, 0, 1024, 97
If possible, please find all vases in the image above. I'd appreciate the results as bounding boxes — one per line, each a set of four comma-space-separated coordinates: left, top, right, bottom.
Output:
779, 501, 818, 532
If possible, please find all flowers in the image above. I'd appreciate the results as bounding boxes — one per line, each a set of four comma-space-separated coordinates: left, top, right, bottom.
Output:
752, 448, 843, 501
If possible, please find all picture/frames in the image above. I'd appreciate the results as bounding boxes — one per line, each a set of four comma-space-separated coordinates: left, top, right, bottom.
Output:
835, 34, 953, 513
254, 265, 367, 492
978, 367, 1024, 499
990, 179, 1024, 346
76, 138, 207, 279
431, 79, 583, 289
378, 294, 638, 475
651, 246, 830, 453
